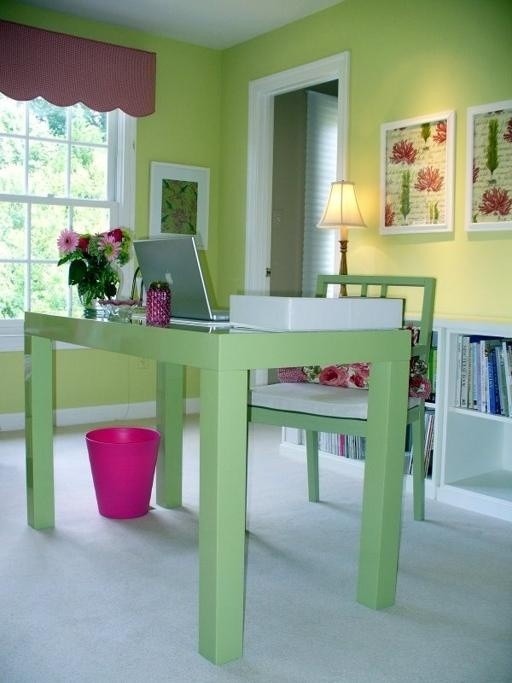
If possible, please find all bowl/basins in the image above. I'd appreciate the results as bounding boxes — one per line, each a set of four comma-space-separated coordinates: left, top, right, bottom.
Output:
98, 299, 139, 316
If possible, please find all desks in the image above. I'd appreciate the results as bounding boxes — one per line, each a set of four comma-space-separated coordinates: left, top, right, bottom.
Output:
22, 309, 412, 662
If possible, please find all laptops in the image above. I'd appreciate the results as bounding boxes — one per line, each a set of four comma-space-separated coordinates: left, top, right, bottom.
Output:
132, 236, 229, 322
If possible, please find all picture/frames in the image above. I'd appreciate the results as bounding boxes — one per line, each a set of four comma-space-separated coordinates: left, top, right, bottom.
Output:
379, 108, 456, 234
466, 99, 512, 234
150, 157, 212, 251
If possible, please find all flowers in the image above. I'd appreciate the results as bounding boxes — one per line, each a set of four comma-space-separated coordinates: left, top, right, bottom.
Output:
56, 227, 132, 280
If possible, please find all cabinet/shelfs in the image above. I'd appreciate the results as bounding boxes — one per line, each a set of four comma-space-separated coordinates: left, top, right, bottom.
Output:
439, 322, 512, 522
281, 327, 439, 499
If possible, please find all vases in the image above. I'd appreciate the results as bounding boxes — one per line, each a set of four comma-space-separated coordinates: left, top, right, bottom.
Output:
80, 287, 111, 317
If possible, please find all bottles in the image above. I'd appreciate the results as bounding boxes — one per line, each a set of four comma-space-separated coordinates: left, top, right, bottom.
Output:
146, 280, 171, 325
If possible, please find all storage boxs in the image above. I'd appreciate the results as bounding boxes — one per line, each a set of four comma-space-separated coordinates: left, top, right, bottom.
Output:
226, 292, 402, 327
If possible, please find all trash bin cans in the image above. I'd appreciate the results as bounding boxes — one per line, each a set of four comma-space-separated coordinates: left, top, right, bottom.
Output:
86, 428, 160, 518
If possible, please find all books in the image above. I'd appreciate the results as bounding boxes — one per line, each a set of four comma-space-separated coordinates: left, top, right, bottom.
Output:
316, 324, 510, 489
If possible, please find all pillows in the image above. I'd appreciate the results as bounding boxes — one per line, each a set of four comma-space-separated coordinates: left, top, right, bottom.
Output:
276, 325, 429, 396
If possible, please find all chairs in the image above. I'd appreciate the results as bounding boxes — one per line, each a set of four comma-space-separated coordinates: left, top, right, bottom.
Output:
245, 272, 437, 521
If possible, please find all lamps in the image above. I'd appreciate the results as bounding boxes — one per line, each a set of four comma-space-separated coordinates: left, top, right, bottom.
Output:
316, 179, 365, 298
